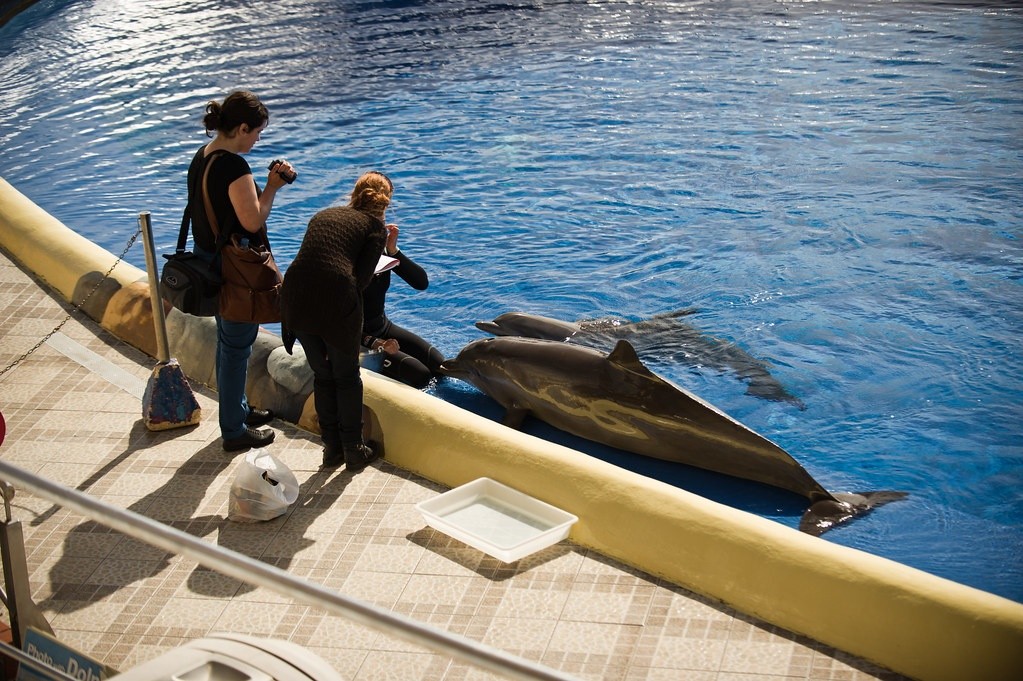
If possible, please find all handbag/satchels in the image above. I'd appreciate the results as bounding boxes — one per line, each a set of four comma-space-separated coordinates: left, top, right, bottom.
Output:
228, 449, 299, 523
217, 245, 283, 324
159, 251, 221, 317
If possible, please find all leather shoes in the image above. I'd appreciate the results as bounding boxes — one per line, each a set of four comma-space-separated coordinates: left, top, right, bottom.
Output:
245, 406, 274, 425
322, 439, 344, 467
342, 439, 380, 471
223, 428, 275, 451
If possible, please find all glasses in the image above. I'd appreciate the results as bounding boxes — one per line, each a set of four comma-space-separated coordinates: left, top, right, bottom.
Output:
364, 171, 393, 196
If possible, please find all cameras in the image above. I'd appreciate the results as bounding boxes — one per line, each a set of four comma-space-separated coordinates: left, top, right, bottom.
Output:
269, 160, 297, 184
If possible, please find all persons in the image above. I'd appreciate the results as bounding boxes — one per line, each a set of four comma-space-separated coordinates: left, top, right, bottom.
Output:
279, 170, 393, 470
187, 91, 295, 452
361, 210, 445, 388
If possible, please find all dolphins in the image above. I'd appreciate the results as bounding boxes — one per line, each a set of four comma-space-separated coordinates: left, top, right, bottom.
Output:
443, 305, 913, 537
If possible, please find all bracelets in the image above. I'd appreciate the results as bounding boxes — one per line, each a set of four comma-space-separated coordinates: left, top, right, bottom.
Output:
391, 249, 399, 256
382, 341, 387, 348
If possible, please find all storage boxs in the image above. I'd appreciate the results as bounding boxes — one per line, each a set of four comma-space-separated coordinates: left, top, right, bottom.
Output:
414, 477, 579, 565
